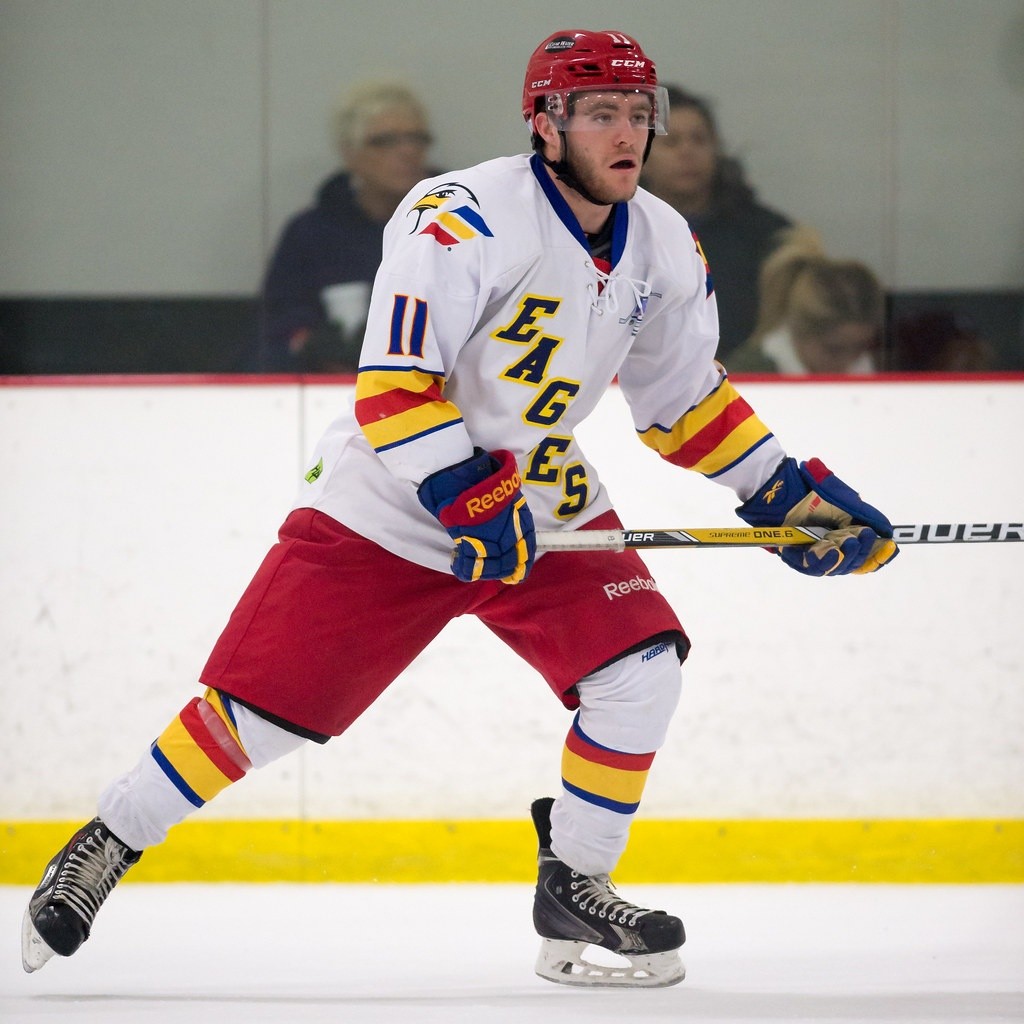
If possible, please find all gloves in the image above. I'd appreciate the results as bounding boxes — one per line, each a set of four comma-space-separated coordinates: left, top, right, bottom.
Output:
735, 456, 900, 578
416, 446, 537, 584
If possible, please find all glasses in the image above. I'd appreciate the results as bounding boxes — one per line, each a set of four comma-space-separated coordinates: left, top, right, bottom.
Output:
367, 132, 433, 150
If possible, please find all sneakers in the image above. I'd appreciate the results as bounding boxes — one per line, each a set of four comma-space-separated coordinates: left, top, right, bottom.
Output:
21, 815, 144, 974
531, 797, 687, 987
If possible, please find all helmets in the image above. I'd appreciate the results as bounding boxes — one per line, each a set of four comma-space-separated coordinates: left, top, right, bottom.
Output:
523, 30, 660, 137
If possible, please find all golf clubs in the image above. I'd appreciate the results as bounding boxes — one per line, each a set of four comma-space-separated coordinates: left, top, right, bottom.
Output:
484, 522, 1024, 552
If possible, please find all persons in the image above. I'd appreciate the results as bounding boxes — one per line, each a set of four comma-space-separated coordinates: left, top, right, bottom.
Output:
18, 31, 900, 988
638, 84, 879, 381
258, 84, 445, 376
